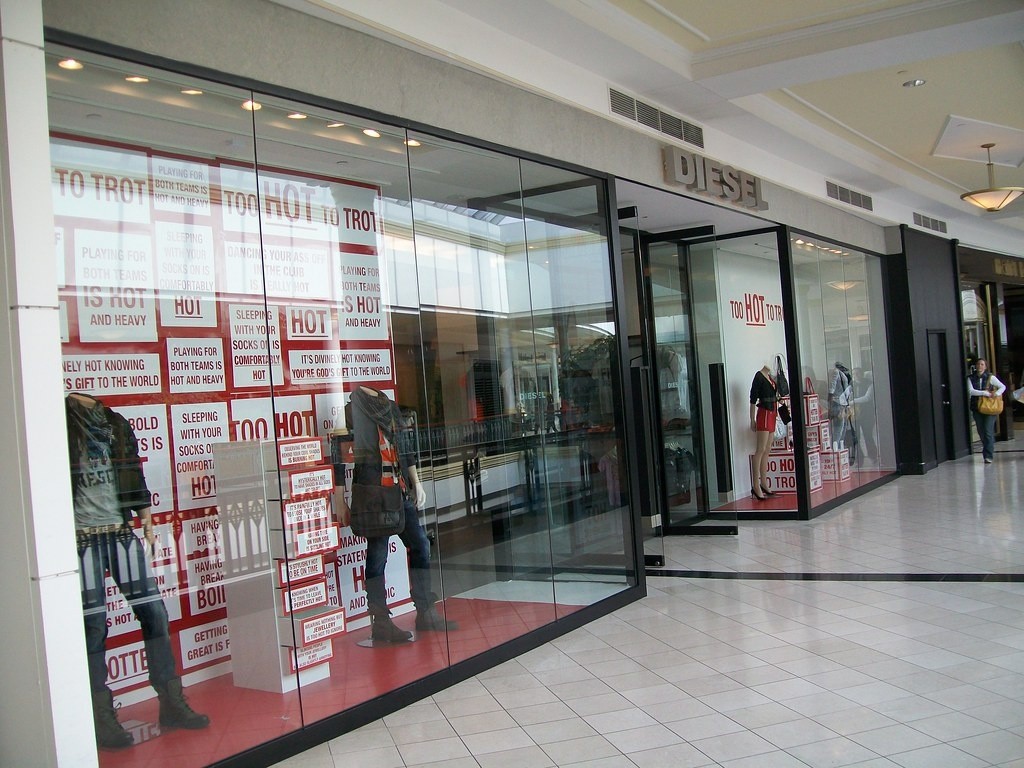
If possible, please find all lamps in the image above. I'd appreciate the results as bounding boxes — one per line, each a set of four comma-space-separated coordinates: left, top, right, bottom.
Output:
826, 271, 856, 291
959, 141, 1024, 212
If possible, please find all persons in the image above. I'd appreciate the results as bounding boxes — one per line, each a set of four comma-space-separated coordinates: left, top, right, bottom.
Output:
828, 361, 853, 449
750, 365, 783, 501
840, 368, 879, 468
967, 359, 1006, 463
330, 385, 458, 642
65, 392, 212, 748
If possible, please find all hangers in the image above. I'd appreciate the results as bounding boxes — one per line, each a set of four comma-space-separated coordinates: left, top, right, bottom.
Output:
610, 436, 684, 454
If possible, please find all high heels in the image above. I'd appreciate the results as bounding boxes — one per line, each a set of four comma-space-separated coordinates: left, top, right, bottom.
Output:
760, 484, 774, 495
751, 486, 767, 500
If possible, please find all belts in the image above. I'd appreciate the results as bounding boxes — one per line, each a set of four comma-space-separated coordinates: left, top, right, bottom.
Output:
759, 397, 777, 402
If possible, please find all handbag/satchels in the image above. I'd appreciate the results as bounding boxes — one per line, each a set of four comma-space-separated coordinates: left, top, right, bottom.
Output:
978, 374, 1004, 415
350, 483, 405, 537
778, 398, 792, 425
1012, 387, 1024, 403
844, 415, 858, 447
777, 359, 789, 397
806, 377, 822, 418
828, 401, 842, 417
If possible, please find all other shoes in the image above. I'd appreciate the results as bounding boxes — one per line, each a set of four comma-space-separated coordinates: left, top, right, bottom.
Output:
985, 457, 992, 463
853, 460, 865, 468
869, 455, 877, 464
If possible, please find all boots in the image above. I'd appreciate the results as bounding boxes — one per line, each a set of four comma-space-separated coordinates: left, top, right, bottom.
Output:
157, 674, 210, 728
92, 687, 135, 747
413, 590, 459, 631
367, 596, 413, 642
